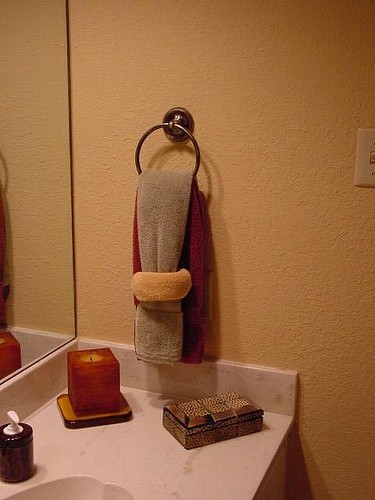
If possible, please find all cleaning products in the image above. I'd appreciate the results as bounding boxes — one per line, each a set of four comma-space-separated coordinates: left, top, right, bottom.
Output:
0, 410, 35, 483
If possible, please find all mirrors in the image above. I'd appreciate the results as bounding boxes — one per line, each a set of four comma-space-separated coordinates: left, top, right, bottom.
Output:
0, 0, 80, 385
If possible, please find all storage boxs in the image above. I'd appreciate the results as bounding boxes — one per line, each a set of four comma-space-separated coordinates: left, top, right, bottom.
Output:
163, 390, 265, 450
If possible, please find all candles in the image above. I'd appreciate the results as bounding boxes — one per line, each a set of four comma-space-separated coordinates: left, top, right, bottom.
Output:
1, 328, 21, 381
65, 344, 123, 417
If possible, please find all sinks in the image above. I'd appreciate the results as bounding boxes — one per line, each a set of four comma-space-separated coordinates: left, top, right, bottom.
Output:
0, 475, 134, 500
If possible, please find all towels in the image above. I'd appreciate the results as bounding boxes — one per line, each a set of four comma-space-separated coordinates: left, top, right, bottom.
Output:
132, 171, 206, 365
